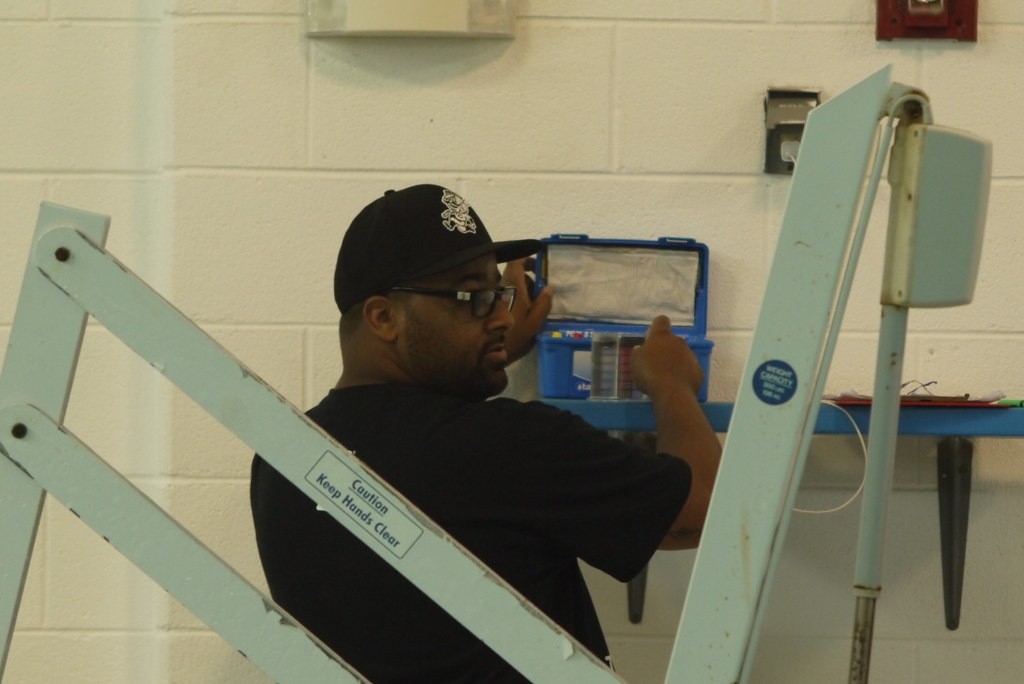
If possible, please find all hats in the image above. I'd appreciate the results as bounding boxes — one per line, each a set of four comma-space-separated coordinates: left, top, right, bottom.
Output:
334, 184, 543, 313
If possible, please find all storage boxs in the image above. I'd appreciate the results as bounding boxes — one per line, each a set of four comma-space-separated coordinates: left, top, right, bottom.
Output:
488, 232, 715, 401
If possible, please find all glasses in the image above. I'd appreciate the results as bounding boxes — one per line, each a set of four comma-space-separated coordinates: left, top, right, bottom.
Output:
386, 285, 518, 320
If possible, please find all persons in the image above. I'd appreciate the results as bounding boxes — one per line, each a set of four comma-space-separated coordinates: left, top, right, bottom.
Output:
250, 183, 722, 684
